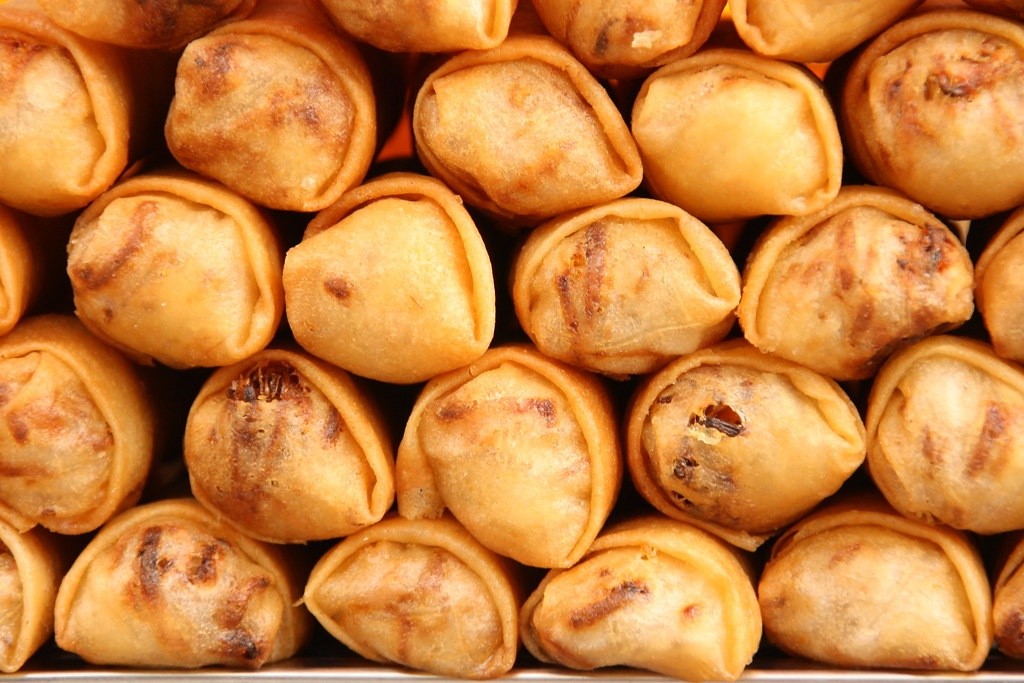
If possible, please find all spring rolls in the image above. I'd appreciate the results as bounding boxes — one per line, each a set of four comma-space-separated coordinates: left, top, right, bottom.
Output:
2, 1, 1024, 682
